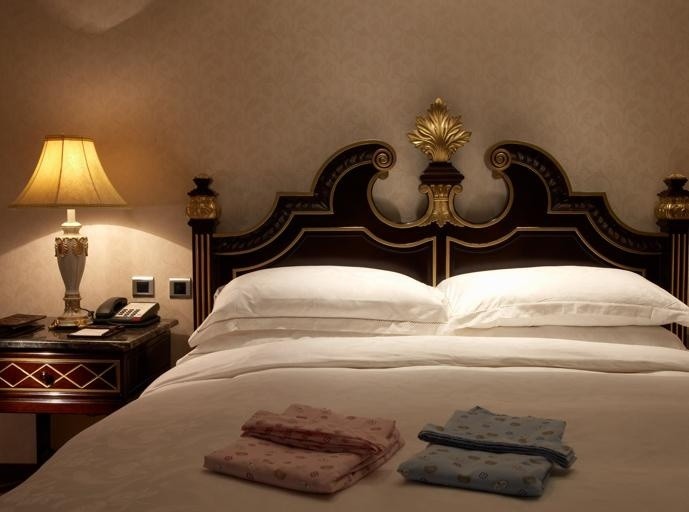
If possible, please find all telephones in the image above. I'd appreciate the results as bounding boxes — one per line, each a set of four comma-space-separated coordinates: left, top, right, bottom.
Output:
87, 297, 160, 327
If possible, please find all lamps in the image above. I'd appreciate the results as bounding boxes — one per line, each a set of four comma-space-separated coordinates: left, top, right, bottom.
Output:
7, 135, 132, 330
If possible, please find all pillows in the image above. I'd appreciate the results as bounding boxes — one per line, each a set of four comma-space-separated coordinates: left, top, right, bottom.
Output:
188, 263, 451, 348
434, 263, 688, 334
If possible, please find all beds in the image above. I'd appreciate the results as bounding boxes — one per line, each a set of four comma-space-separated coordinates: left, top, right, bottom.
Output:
1, 95, 687, 511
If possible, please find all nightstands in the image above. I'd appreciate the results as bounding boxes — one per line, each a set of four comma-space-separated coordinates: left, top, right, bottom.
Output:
0, 313, 178, 469
